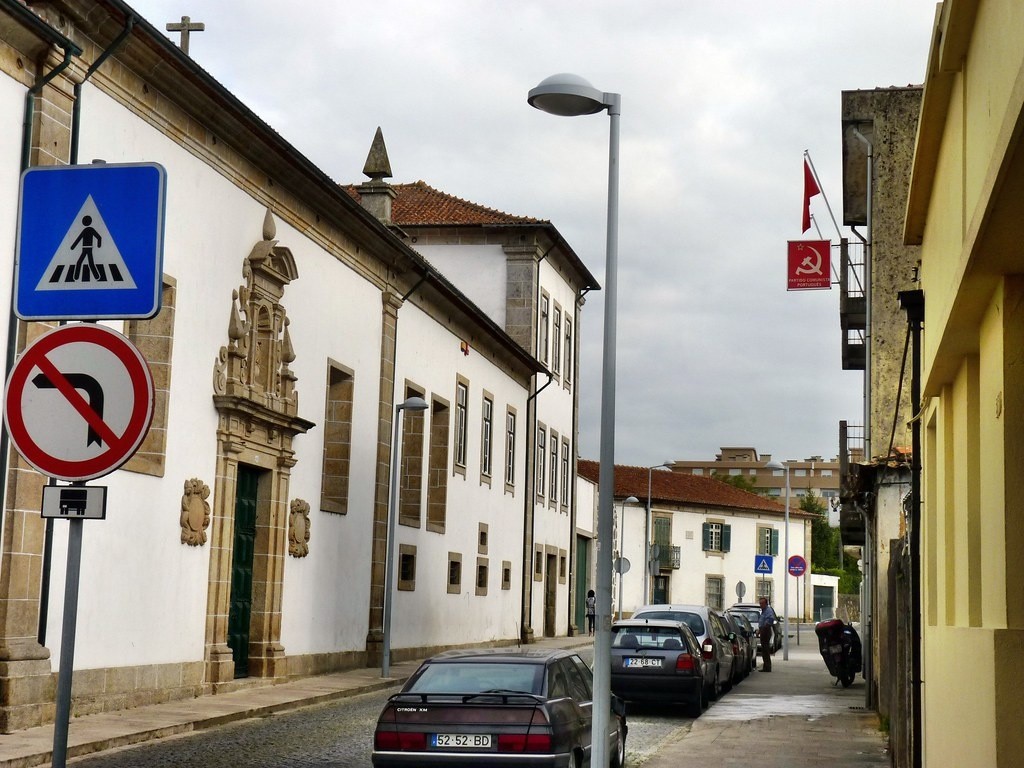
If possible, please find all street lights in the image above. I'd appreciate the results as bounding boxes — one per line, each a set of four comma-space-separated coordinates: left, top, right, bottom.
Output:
380, 397, 429, 678
643, 459, 677, 606
527, 73, 622, 768
614, 496, 639, 621
763, 459, 790, 660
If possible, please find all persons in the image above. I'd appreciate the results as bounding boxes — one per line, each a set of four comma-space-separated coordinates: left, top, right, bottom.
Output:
758, 598, 774, 672
586, 590, 596, 637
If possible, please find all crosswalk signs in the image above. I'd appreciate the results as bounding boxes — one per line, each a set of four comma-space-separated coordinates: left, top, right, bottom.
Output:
754, 555, 774, 574
15, 166, 162, 316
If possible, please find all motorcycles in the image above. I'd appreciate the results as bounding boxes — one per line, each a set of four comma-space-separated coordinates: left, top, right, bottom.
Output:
814, 604, 862, 689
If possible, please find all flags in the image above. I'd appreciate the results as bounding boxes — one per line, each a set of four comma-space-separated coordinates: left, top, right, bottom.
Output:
802, 159, 820, 233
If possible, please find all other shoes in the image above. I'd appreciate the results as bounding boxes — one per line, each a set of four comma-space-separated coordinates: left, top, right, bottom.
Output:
758, 669, 770, 672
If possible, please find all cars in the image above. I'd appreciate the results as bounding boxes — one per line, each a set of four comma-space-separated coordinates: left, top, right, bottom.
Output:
723, 603, 785, 653
590, 604, 759, 720
371, 648, 628, 767
